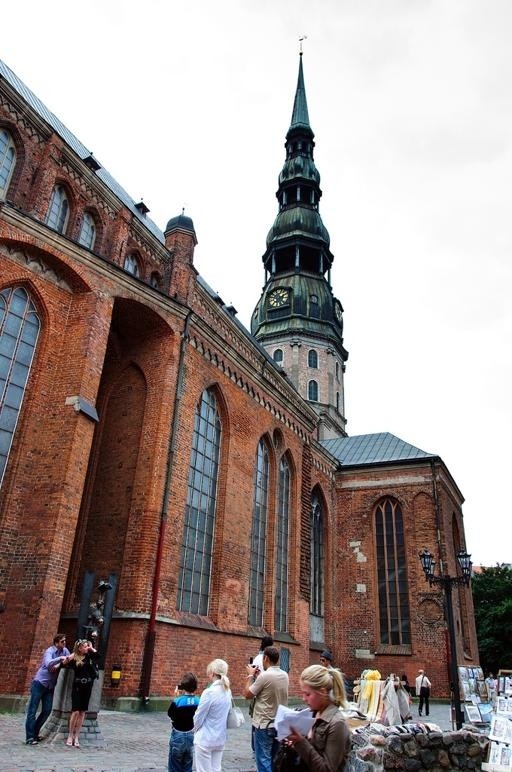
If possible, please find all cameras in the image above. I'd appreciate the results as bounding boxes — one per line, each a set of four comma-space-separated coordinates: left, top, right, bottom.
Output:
250, 664, 259, 668
176, 685, 183, 691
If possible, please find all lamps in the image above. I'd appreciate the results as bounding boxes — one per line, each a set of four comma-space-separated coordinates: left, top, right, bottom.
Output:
110, 665, 122, 687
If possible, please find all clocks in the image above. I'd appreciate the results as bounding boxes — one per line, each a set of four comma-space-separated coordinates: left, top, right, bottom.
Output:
334, 301, 345, 326
268, 286, 291, 307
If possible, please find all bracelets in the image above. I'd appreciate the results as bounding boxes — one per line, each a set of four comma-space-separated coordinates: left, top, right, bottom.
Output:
247, 675, 254, 678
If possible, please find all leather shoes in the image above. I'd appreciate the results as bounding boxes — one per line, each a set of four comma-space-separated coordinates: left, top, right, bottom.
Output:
66, 741, 80, 747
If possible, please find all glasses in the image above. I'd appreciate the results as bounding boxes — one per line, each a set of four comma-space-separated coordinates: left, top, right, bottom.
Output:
61, 639, 67, 644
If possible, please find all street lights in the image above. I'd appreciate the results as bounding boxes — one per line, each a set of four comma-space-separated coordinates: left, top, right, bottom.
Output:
416, 545, 474, 731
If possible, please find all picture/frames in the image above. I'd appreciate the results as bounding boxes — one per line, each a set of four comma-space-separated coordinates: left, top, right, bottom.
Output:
460, 666, 510, 726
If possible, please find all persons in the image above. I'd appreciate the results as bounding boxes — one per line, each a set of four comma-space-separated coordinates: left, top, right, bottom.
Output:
416, 670, 432, 716
192, 657, 231, 772
21, 634, 69, 744
247, 636, 273, 717
51, 639, 100, 747
401, 674, 413, 722
273, 663, 353, 772
243, 646, 290, 771
319, 650, 334, 671
486, 673, 497, 699
166, 672, 199, 772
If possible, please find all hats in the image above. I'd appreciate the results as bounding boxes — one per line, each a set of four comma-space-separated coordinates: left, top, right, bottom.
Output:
321, 650, 331, 660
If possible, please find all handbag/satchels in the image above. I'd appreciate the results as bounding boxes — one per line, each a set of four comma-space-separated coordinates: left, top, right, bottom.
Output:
228, 698, 245, 730
420, 686, 429, 698
265, 719, 299, 772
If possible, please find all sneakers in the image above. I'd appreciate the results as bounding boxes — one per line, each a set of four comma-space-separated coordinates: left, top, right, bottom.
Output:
29, 740, 38, 745
34, 736, 41, 742
